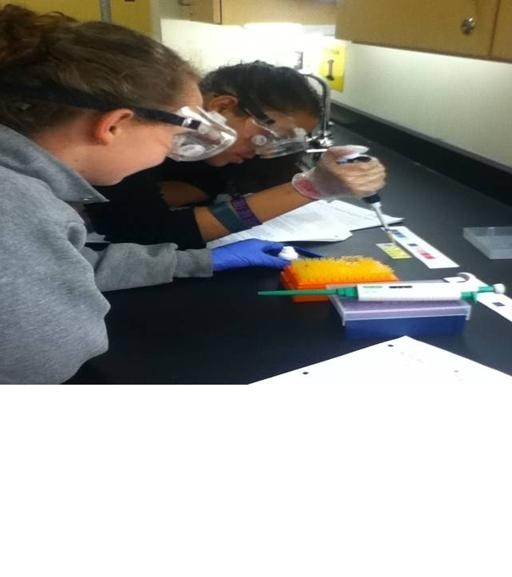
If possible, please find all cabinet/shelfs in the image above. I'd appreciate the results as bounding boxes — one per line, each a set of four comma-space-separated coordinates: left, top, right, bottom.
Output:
335, 0, 512, 62
179, 0, 335, 26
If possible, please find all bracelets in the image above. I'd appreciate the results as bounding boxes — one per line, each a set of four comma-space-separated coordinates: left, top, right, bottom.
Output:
231, 196, 264, 226
210, 202, 242, 233
292, 172, 321, 199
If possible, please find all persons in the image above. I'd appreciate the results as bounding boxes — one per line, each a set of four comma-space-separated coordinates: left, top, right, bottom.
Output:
1, 3, 290, 385
78, 61, 385, 385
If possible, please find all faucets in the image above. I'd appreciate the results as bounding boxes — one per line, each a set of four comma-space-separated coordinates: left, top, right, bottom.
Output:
294, 68, 335, 149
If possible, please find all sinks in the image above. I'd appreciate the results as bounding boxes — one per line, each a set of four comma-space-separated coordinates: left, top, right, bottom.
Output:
222, 163, 311, 192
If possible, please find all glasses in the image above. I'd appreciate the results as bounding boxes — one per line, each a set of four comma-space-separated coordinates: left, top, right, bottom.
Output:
165, 103, 238, 165
244, 110, 314, 163
